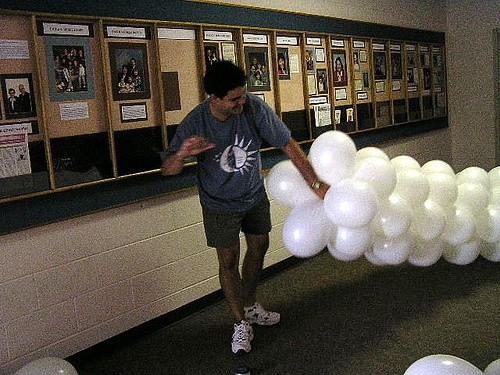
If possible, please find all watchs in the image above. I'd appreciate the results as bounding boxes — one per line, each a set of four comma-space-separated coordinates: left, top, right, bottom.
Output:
310, 180, 324, 190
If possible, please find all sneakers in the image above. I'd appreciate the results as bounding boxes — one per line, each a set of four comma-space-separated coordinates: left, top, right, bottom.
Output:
241, 301, 280, 326
231, 319, 254, 355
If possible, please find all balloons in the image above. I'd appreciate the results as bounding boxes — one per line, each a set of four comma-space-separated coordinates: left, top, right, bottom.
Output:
404, 353, 500, 375
13, 357, 79, 375
266, 130, 500, 265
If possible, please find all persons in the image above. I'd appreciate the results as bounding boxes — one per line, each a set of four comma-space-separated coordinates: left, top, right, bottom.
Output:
250, 57, 268, 85
306, 50, 313, 69
206, 49, 218, 65
56, 49, 87, 92
335, 57, 345, 85
160, 60, 331, 356
119, 59, 145, 92
318, 71, 326, 91
6, 83, 33, 114
278, 54, 288, 75
